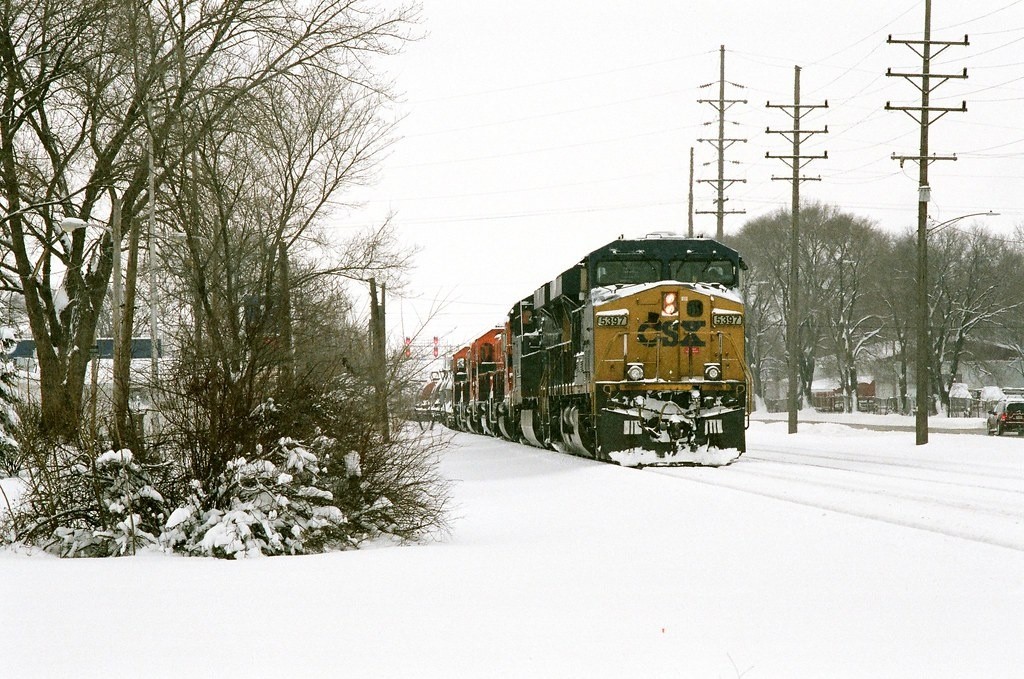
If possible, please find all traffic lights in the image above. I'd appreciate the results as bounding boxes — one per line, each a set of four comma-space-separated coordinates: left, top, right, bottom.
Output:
433, 337, 439, 357
405, 336, 410, 357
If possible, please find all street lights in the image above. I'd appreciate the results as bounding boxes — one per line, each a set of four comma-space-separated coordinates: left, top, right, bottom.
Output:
60, 197, 123, 420
917, 211, 1002, 449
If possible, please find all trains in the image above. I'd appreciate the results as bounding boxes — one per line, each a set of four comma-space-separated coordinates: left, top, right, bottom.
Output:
396, 231, 752, 468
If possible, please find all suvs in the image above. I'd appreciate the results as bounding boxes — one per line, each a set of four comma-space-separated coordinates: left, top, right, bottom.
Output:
986, 400, 1024, 436
